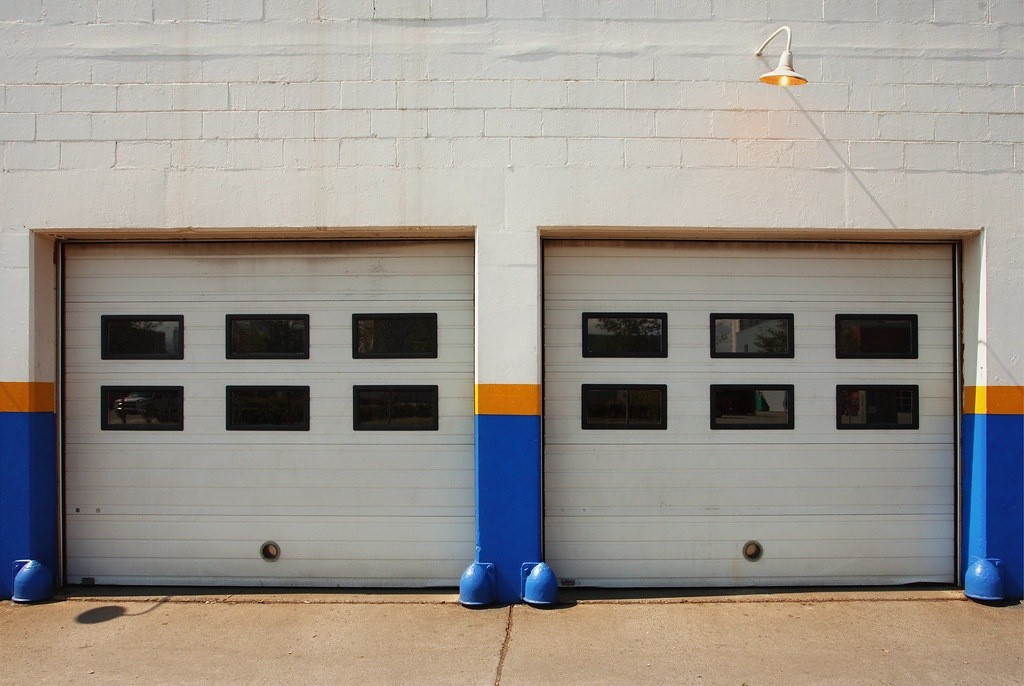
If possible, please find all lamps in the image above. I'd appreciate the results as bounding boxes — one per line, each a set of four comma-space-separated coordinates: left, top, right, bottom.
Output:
754, 26, 808, 86
